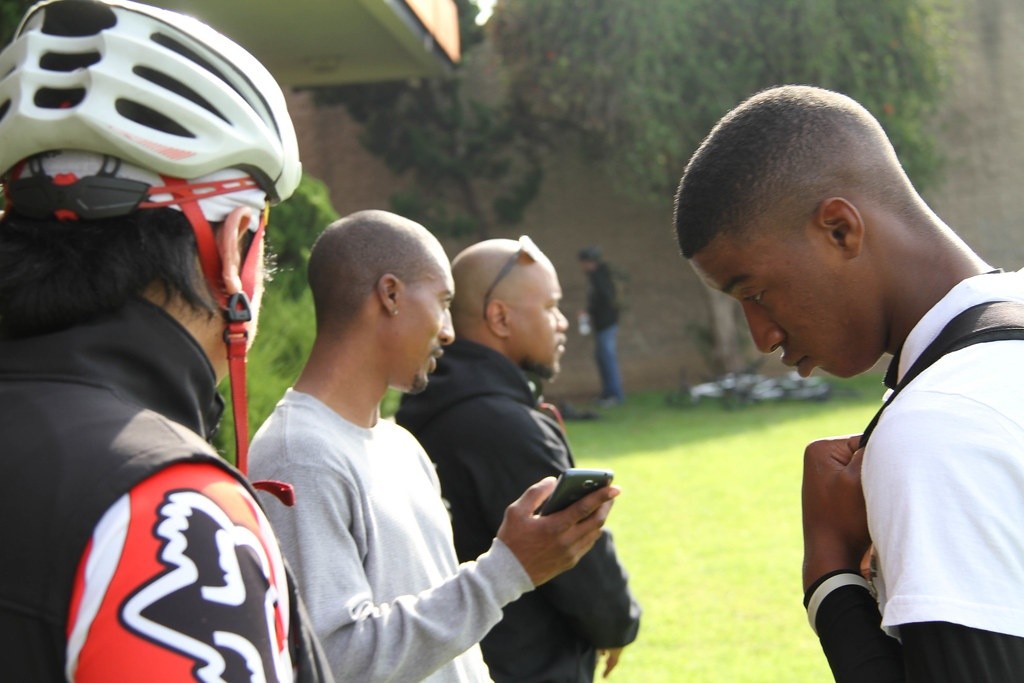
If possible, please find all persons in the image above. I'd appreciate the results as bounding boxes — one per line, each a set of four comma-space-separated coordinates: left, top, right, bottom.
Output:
576, 247, 625, 408
0, 0, 333, 683
673, 84, 1023, 683
246, 209, 621, 683
393, 235, 642, 683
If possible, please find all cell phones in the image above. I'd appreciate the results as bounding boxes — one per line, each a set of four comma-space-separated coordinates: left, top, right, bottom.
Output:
539, 469, 614, 517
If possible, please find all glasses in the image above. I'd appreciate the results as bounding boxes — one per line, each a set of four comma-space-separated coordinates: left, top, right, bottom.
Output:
483, 235, 543, 320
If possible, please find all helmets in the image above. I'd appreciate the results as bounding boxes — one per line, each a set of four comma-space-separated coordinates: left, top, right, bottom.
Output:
0, 0, 304, 221
